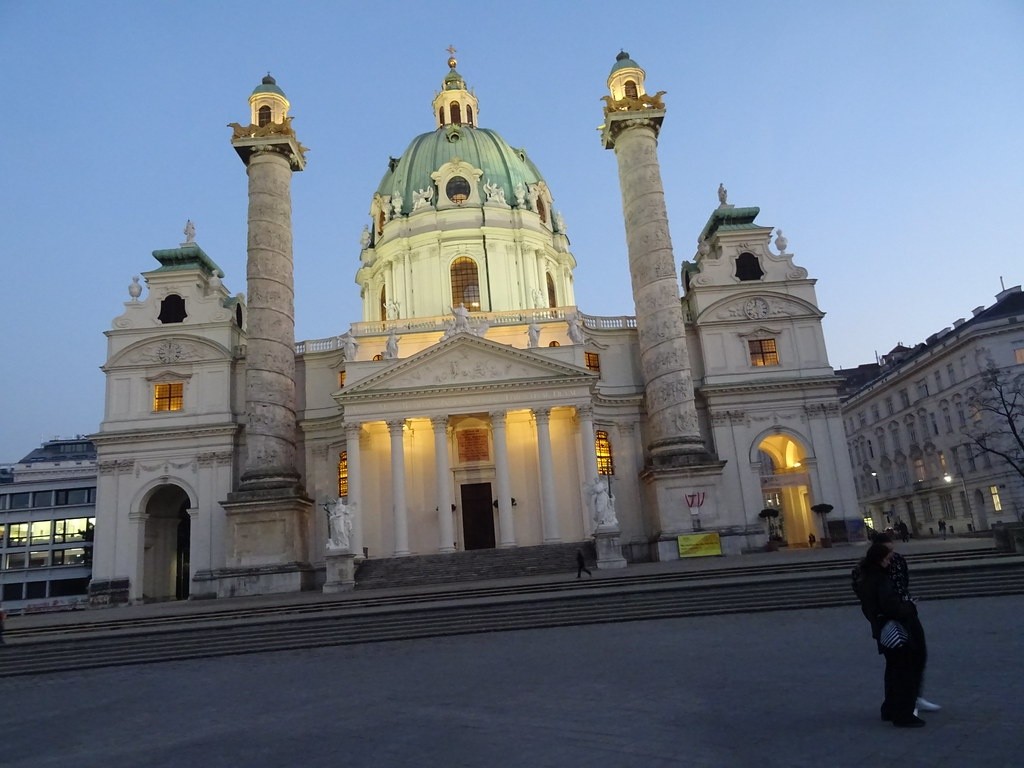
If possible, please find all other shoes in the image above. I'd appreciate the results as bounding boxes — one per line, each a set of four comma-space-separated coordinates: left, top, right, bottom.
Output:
881, 711, 892, 722
913, 697, 942, 716
892, 714, 926, 727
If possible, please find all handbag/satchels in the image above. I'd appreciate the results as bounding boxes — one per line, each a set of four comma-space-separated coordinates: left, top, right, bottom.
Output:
881, 619, 909, 648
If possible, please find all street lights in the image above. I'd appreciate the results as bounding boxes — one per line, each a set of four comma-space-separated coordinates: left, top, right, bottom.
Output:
944, 463, 977, 534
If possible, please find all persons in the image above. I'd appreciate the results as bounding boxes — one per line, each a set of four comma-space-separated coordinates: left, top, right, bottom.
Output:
384, 329, 402, 359
893, 522, 901, 539
899, 520, 909, 543
590, 477, 615, 526
565, 314, 585, 345
337, 331, 359, 361
718, 183, 728, 204
851, 532, 943, 717
808, 533, 815, 547
524, 321, 547, 348
939, 518, 947, 534
361, 182, 568, 248
576, 548, 592, 578
451, 302, 470, 334
329, 498, 352, 547
857, 544, 926, 727
185, 220, 196, 244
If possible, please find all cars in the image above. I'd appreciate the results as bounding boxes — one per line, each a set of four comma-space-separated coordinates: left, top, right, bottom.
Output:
884, 526, 910, 540
